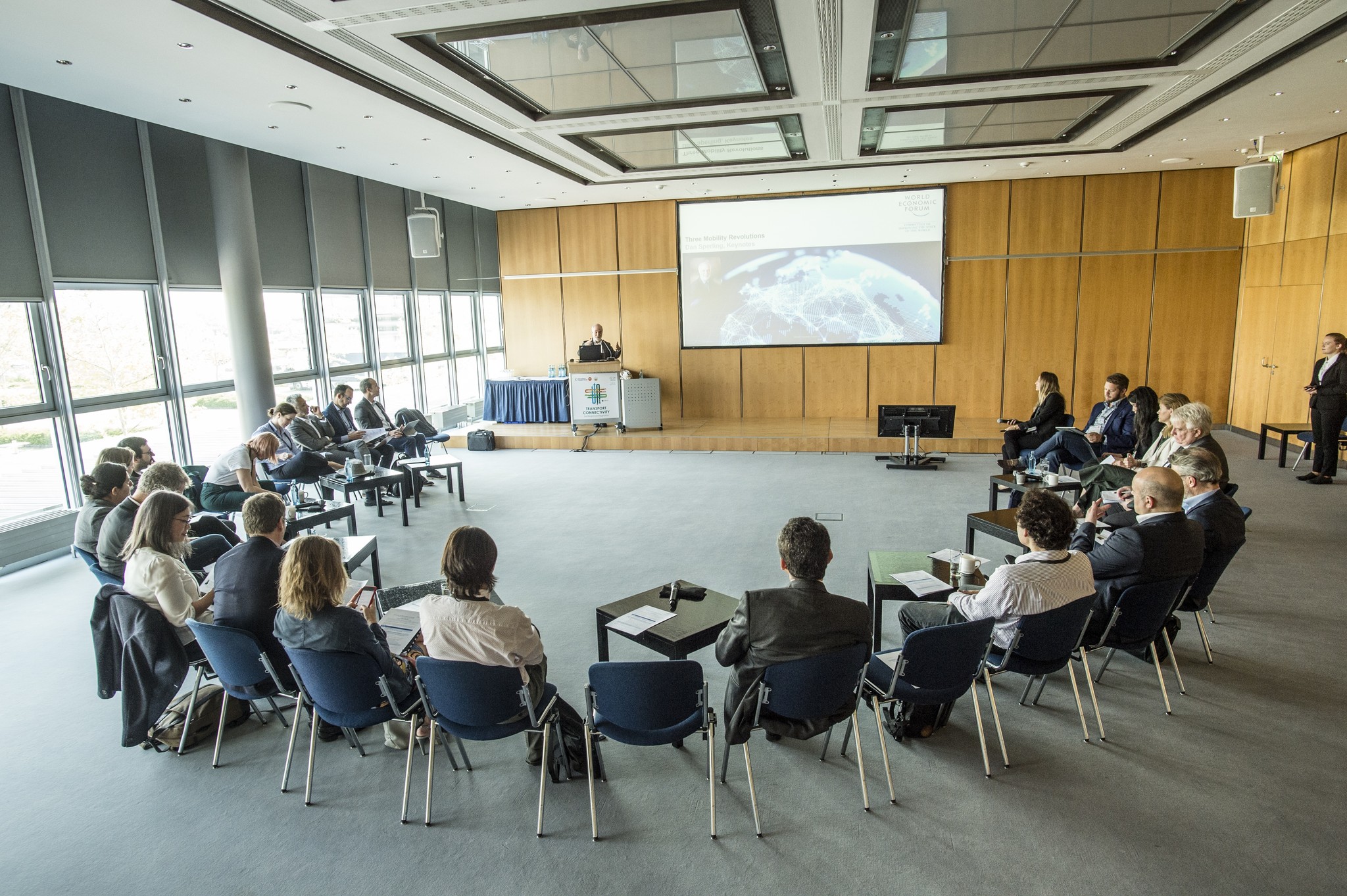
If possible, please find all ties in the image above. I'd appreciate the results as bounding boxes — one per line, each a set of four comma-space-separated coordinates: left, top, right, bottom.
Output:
1163, 447, 1183, 469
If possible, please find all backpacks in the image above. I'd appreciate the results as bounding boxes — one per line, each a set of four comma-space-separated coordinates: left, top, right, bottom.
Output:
142, 684, 251, 753
386, 452, 424, 498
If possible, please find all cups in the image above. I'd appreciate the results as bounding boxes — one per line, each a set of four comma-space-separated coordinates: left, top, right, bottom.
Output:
317, 533, 327, 538
950, 548, 962, 577
298, 490, 308, 502
514, 377, 521, 380
1016, 473, 1026, 484
441, 583, 451, 596
285, 505, 296, 519
1041, 461, 1050, 477
364, 454, 371, 465
959, 555, 981, 575
1040, 458, 1048, 474
1045, 474, 1059, 486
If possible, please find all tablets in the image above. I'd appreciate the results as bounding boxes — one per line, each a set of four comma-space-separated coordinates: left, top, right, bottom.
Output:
1055, 426, 1086, 435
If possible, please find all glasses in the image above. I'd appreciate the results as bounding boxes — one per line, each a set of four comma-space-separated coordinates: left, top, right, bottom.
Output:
339, 392, 353, 402
172, 516, 192, 528
282, 517, 289, 528
141, 450, 152, 456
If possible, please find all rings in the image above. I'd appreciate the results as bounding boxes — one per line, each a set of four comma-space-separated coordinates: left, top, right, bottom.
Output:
1115, 463, 1117, 465
361, 436, 361, 438
285, 457, 287, 459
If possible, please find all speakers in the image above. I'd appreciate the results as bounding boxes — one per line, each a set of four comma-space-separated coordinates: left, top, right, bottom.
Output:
408, 214, 441, 258
1232, 162, 1278, 218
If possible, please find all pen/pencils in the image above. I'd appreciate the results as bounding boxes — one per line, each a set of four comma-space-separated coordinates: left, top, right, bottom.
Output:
1112, 491, 1131, 494
1127, 453, 1132, 470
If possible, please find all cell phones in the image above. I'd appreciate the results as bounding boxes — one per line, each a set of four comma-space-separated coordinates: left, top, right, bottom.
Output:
1304, 385, 1317, 390
354, 586, 378, 612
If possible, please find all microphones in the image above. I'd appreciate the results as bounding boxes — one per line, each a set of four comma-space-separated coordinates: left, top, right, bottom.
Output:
997, 419, 1019, 424
295, 499, 324, 508
602, 340, 615, 361
669, 581, 681, 606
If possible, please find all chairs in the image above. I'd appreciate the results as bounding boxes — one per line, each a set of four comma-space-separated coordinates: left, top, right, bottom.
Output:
72, 415, 1252, 838
1293, 417, 1347, 472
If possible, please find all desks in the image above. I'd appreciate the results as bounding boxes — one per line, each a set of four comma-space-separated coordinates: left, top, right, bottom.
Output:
375, 579, 505, 623
285, 498, 357, 539
483, 376, 570, 424
319, 465, 408, 535
1258, 423, 1313, 467
966, 507, 1078, 555
401, 454, 465, 508
597, 579, 739, 662
280, 535, 382, 609
989, 471, 1081, 513
867, 550, 986, 652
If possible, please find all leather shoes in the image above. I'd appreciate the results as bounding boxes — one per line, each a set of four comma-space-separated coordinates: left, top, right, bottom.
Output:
365, 497, 393, 507
426, 470, 447, 480
1004, 555, 1017, 565
420, 476, 434, 486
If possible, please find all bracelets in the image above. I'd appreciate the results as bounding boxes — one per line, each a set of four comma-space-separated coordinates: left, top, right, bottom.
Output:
1138, 460, 1142, 467
1014, 425, 1017, 430
1135, 459, 1139, 467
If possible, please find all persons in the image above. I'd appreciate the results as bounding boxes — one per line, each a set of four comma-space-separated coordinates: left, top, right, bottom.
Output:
251, 402, 344, 502
1296, 332, 1347, 484
577, 324, 621, 428
353, 378, 448, 486
123, 489, 215, 674
714, 517, 874, 743
988, 371, 1065, 493
996, 373, 1137, 475
898, 489, 1096, 681
284, 394, 394, 506
1071, 385, 1245, 612
213, 492, 365, 742
199, 431, 280, 514
74, 435, 244, 578
983, 466, 1206, 647
273, 535, 446, 755
418, 526, 547, 767
322, 384, 394, 497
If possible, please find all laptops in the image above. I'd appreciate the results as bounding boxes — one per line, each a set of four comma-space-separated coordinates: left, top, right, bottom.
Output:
401, 419, 420, 434
578, 345, 601, 363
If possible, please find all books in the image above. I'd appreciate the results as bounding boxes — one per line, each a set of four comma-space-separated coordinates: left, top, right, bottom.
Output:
1095, 529, 1112, 545
1055, 426, 1088, 435
1100, 490, 1126, 504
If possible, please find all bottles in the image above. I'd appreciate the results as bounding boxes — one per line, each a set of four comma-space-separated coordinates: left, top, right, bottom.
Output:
558, 364, 566, 377
1029, 451, 1036, 474
548, 365, 555, 378
291, 479, 300, 505
639, 369, 643, 379
424, 444, 430, 464
345, 457, 353, 481
310, 528, 316, 535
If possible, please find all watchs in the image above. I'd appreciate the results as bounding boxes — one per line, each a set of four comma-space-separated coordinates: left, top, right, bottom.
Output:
318, 415, 325, 420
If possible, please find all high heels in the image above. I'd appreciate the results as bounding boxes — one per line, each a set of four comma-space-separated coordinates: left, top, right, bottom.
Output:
415, 724, 447, 755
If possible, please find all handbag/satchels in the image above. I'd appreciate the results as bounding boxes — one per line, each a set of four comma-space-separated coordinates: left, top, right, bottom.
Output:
549, 694, 601, 778
1124, 613, 1181, 664
383, 717, 426, 750
467, 429, 495, 451
881, 700, 956, 742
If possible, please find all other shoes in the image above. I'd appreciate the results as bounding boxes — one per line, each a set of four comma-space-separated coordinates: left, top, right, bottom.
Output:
1306, 475, 1333, 484
594, 423, 599, 427
362, 490, 385, 497
1296, 472, 1319, 481
600, 423, 607, 427
996, 458, 1029, 471
988, 486, 1012, 493
308, 718, 321, 732
525, 759, 540, 766
317, 725, 366, 743
